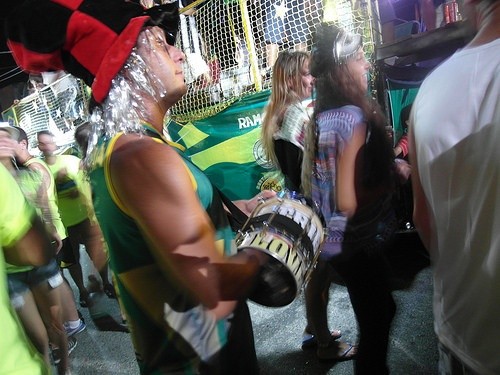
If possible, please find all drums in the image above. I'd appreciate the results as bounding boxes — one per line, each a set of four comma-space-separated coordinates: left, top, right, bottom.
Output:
234, 193, 325, 308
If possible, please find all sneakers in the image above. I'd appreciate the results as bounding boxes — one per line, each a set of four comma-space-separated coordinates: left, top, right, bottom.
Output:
76, 282, 118, 306
61, 320, 86, 334
51, 338, 78, 363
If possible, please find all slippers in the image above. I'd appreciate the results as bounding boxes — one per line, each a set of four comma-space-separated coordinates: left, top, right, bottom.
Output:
301, 328, 358, 360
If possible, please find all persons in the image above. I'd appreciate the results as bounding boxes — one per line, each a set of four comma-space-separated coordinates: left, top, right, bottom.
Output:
261, 51, 357, 363
264, 0, 357, 80
407, 0, 500, 375
301, 25, 431, 375
0, 0, 279, 375
0, 123, 87, 375
38, 132, 118, 307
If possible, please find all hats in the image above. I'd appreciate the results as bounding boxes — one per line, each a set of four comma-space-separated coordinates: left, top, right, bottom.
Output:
3, 0, 180, 112
